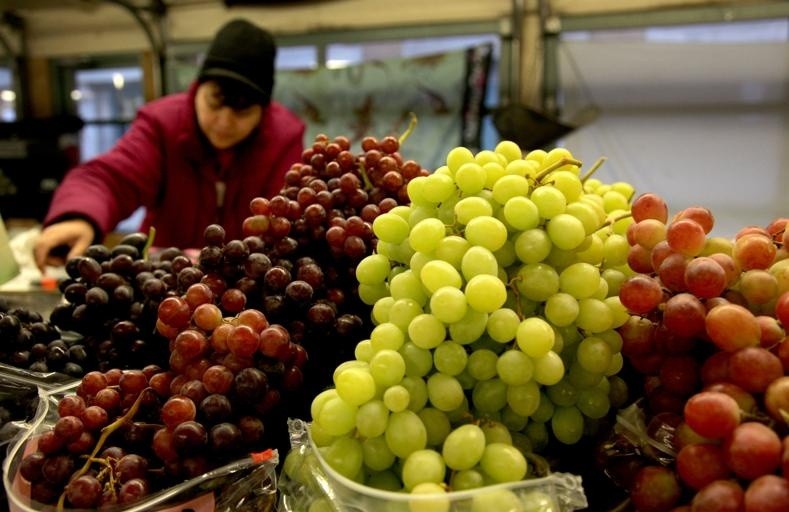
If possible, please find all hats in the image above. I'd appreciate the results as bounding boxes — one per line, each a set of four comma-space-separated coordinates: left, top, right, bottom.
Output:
198, 20, 275, 104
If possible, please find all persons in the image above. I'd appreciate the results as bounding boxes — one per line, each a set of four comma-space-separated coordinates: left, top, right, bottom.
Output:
34, 18, 305, 273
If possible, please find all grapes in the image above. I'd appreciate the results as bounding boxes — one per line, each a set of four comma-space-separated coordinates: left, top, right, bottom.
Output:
0, 133, 789, 512
